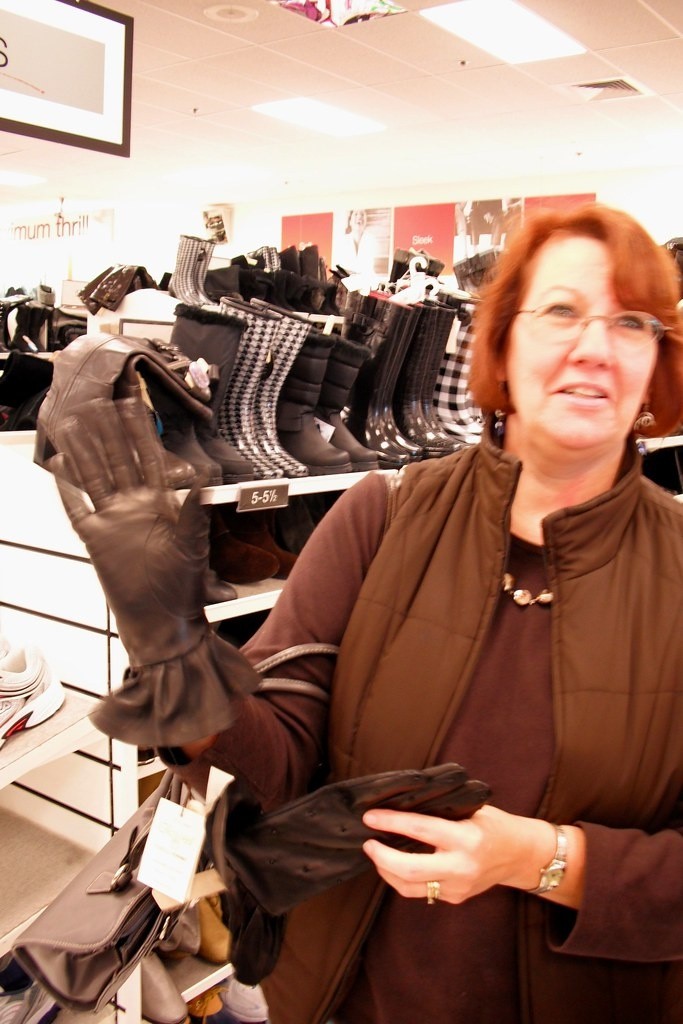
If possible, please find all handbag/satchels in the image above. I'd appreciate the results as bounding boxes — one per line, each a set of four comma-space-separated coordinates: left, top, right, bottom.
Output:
10, 767, 209, 1012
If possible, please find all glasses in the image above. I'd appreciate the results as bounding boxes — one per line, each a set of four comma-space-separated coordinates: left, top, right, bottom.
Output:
517, 300, 672, 345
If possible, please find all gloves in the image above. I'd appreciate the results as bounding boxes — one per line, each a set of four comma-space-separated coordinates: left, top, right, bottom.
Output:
216, 764, 492, 988
47, 397, 261, 749
214, 796, 284, 982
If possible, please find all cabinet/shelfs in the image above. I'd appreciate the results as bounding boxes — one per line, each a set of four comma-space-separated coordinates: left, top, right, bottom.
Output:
0, 288, 683, 1024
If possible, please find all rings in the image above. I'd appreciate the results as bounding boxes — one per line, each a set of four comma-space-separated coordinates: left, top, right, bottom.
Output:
426, 881, 440, 904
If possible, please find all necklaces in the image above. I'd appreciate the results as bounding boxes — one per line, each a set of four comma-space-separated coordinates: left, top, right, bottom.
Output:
502, 574, 553, 606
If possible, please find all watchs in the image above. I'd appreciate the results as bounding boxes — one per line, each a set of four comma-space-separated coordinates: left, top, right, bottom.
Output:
529, 823, 567, 894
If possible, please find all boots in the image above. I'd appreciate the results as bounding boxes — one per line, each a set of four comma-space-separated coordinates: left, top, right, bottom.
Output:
0, 236, 472, 485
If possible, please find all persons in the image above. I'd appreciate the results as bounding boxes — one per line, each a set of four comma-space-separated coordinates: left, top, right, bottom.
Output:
49, 204, 683, 1024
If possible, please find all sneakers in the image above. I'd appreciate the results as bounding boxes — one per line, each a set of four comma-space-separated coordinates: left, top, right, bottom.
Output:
0, 649, 62, 756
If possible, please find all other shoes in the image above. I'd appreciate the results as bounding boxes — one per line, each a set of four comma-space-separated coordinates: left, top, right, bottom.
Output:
141, 953, 186, 1024
189, 986, 224, 1024
221, 980, 269, 1023
195, 489, 338, 602
